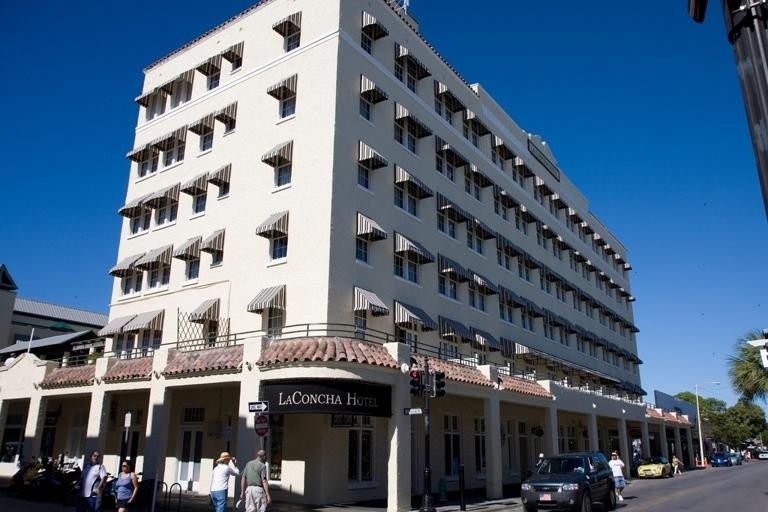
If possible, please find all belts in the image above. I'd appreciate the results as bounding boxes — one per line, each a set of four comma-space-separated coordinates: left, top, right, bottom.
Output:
250, 485, 263, 487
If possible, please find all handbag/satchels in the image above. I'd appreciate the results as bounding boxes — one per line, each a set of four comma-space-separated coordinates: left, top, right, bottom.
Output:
91, 476, 111, 496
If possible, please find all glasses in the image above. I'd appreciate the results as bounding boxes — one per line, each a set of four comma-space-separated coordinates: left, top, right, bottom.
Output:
122, 465, 128, 467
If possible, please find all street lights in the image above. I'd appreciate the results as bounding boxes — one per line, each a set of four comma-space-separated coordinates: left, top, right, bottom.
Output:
695, 382, 720, 467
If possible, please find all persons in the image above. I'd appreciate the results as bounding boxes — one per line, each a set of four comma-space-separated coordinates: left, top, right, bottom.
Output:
535, 453, 544, 467
588, 457, 599, 470
240, 450, 273, 512
79, 450, 108, 512
608, 451, 626, 501
671, 455, 684, 476
209, 451, 240, 512
114, 460, 139, 512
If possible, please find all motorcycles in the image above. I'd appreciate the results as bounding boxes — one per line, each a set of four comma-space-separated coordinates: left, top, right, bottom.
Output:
11, 454, 80, 501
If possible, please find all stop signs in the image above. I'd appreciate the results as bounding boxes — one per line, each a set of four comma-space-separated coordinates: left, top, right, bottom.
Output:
255, 416, 268, 436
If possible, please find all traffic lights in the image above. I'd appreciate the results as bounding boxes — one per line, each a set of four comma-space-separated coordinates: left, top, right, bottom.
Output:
432, 371, 445, 398
409, 371, 423, 395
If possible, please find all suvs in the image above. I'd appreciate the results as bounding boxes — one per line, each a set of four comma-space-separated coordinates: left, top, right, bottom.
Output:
521, 452, 616, 511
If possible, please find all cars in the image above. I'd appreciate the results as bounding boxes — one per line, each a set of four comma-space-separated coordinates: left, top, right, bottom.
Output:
637, 456, 672, 478
711, 453, 742, 466
758, 451, 768, 459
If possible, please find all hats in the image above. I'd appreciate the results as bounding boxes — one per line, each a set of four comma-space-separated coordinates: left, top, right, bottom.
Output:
217, 452, 232, 461
